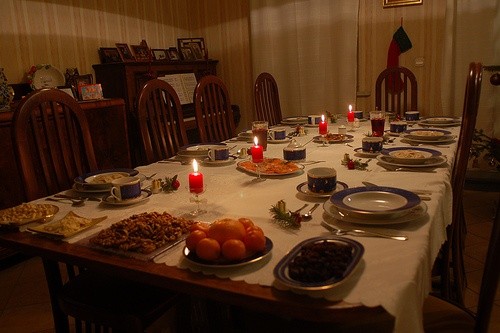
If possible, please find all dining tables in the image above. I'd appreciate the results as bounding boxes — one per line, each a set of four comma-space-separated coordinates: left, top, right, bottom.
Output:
0, 111, 467, 333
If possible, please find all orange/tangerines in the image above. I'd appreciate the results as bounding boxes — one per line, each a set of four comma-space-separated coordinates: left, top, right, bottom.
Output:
186, 218, 266, 260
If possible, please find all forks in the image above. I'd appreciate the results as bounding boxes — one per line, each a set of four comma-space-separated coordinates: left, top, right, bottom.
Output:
378, 162, 437, 173
320, 221, 408, 240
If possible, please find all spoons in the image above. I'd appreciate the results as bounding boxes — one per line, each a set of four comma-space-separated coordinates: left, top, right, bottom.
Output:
363, 182, 432, 194
159, 160, 191, 165
302, 204, 319, 219
46, 198, 88, 206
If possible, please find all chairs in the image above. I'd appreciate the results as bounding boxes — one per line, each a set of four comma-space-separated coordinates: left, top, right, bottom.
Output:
136, 79, 189, 163
254, 72, 282, 128
193, 75, 236, 144
375, 66, 418, 118
422, 61, 500, 333
11, 88, 192, 332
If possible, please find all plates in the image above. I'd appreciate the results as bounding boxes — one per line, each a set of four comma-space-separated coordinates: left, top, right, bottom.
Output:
0, 204, 59, 224
405, 135, 453, 142
377, 154, 447, 166
77, 213, 192, 260
73, 168, 155, 192
421, 116, 459, 123
419, 121, 460, 126
323, 199, 428, 224
314, 134, 353, 142
304, 124, 319, 127
273, 236, 364, 291
330, 186, 421, 214
238, 129, 252, 140
102, 189, 152, 204
204, 157, 234, 163
237, 157, 305, 175
177, 142, 238, 158
354, 148, 386, 155
267, 137, 289, 142
402, 119, 421, 122
406, 129, 451, 139
27, 210, 107, 236
359, 118, 367, 122
281, 116, 308, 124
385, 130, 410, 136
382, 147, 442, 162
297, 181, 348, 197
183, 235, 273, 267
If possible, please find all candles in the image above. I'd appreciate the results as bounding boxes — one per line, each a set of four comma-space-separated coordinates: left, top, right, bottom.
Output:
347, 105, 354, 122
187, 159, 204, 193
319, 115, 328, 134
251, 136, 263, 163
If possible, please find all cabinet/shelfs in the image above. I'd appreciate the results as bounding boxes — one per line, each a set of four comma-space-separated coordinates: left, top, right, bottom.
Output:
0, 97, 133, 270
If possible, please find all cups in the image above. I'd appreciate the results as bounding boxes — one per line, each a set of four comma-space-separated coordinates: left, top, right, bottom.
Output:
283, 148, 306, 161
307, 168, 336, 193
308, 115, 321, 125
269, 128, 285, 140
208, 147, 229, 161
362, 137, 383, 152
405, 111, 419, 120
369, 111, 385, 137
354, 111, 363, 119
252, 121, 268, 151
390, 122, 407, 133
111, 177, 141, 201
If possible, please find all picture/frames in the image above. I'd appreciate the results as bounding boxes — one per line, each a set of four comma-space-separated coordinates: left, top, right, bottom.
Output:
54, 74, 93, 102
99, 37, 207, 62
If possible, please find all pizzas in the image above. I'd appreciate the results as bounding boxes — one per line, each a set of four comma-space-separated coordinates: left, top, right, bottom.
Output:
239, 158, 301, 174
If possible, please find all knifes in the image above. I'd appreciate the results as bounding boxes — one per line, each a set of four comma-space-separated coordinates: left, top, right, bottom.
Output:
54, 194, 101, 202
